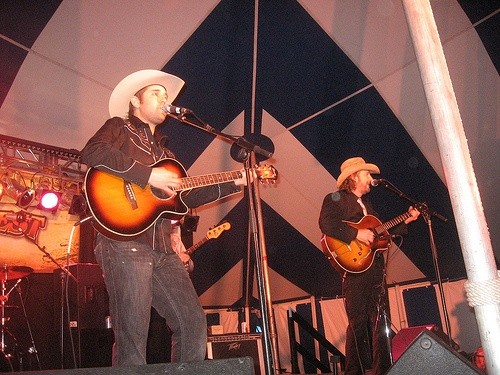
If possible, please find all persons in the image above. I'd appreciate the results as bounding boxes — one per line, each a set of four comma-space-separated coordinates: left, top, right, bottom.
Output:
318, 157, 421, 375
170, 216, 195, 273
78, 69, 257, 367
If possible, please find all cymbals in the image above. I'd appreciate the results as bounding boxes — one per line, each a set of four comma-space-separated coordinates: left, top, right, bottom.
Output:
0, 264, 33, 281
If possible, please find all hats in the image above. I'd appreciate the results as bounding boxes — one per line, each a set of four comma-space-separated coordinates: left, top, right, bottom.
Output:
109, 70, 185, 119
337, 157, 380, 188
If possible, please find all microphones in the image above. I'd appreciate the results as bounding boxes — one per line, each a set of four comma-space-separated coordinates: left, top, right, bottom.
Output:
162, 105, 190, 115
13, 225, 23, 232
371, 179, 383, 186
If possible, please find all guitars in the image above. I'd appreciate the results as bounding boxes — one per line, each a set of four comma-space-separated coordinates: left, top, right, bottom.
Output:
319, 199, 444, 277
83, 163, 295, 243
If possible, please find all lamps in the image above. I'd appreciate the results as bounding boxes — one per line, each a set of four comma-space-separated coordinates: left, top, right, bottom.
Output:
61, 188, 90, 217
3, 178, 36, 209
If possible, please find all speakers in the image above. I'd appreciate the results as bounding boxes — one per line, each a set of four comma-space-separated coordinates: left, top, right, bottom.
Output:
383, 325, 486, 375
206, 332, 265, 375
0, 357, 255, 375
